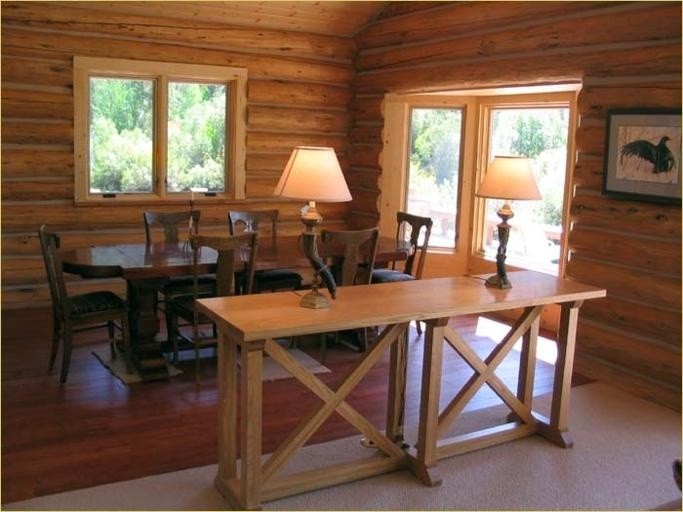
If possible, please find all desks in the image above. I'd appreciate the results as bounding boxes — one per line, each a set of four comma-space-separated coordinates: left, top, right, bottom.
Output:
191, 266, 609, 511
54, 230, 417, 376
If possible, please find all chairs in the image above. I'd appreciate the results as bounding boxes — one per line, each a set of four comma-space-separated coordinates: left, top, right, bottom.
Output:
368, 211, 435, 335
227, 207, 304, 293
140, 209, 215, 318
164, 232, 259, 375
292, 227, 382, 354
35, 222, 139, 385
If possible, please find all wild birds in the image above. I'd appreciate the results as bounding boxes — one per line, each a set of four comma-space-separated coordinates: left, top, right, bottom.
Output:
619, 136, 677, 175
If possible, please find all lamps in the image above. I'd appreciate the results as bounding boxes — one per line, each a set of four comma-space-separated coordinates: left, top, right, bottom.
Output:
269, 143, 357, 312
469, 153, 549, 293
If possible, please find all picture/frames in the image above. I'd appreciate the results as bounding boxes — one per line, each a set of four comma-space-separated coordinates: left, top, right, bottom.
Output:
600, 106, 682, 208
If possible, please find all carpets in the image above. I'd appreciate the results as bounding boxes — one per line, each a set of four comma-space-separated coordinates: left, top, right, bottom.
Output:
1, 375, 681, 511
